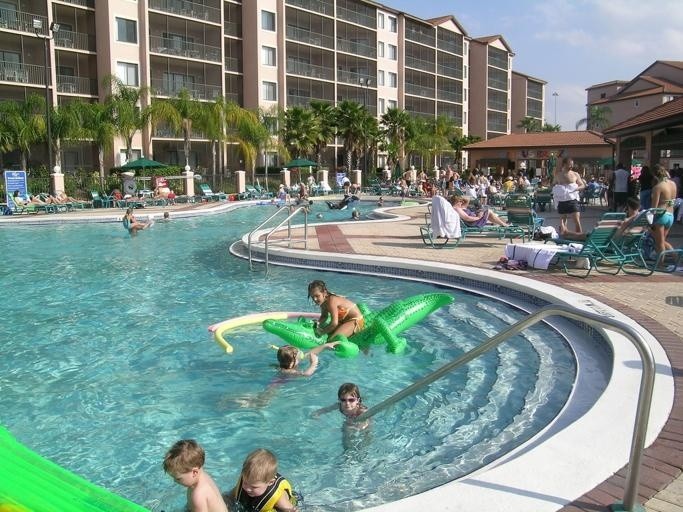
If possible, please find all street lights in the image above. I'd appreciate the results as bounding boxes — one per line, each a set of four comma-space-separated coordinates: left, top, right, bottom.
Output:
359, 77, 372, 184
33, 19, 60, 173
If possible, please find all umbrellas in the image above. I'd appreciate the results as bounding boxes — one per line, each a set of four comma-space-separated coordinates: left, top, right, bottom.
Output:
120, 157, 167, 188
281, 158, 320, 182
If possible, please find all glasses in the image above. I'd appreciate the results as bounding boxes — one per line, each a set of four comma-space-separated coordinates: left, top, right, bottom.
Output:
339, 398, 355, 401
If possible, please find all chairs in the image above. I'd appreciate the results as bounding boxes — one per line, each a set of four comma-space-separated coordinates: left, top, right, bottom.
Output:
420, 192, 553, 248
9, 193, 92, 215
290, 185, 404, 197
545, 208, 683, 276
550, 213, 626, 278
174, 184, 274, 204
90, 192, 169, 209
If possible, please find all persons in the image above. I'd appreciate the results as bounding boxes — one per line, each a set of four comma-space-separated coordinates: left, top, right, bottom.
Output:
393, 158, 682, 268
214, 341, 343, 414
163, 212, 171, 220
269, 173, 392, 220
161, 438, 228, 511
130, 214, 149, 238
309, 382, 375, 453
220, 447, 299, 511
122, 208, 144, 228
107, 186, 124, 207
13, 190, 91, 207
154, 182, 174, 201
305, 280, 364, 340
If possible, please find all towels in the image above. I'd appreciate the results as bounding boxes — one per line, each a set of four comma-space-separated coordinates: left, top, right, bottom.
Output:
570, 243, 590, 270
505, 243, 567, 270
